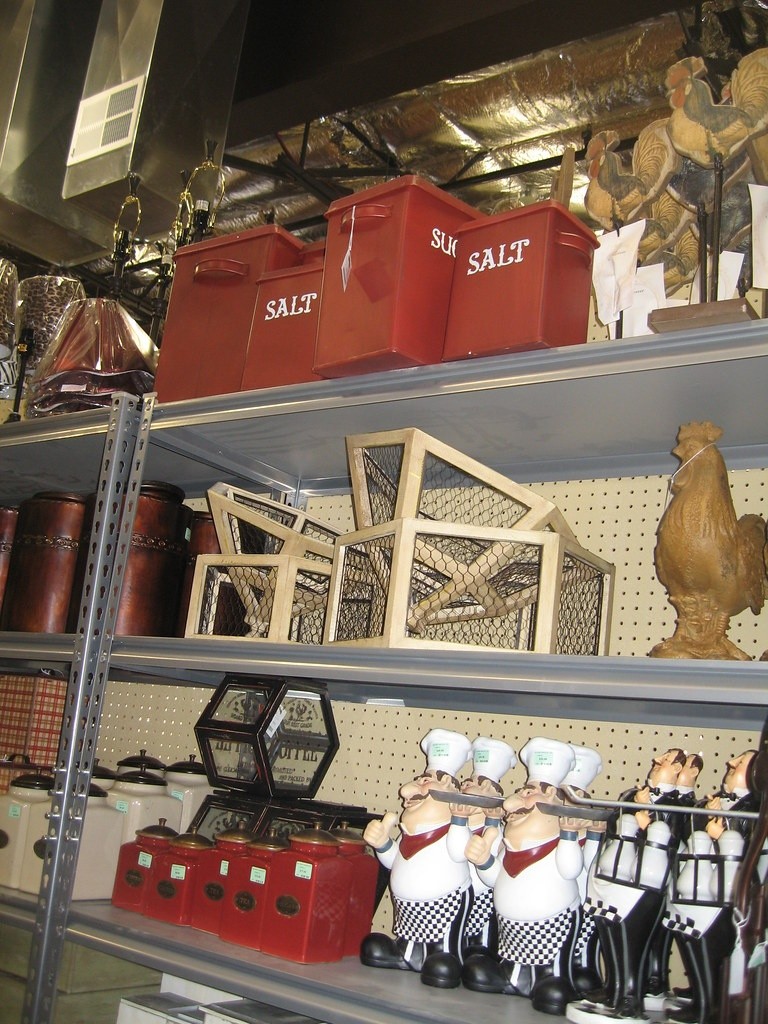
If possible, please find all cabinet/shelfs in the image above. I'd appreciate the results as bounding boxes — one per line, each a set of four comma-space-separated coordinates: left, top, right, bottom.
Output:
32, 320, 768, 1024
0, 391, 287, 1024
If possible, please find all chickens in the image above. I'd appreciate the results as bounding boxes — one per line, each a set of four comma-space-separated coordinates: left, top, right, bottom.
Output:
583, 50, 768, 301
655, 420, 768, 659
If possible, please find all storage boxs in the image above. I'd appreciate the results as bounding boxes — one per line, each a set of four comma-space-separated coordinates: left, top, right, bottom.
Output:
441, 199, 602, 362
312, 173, 489, 379
152, 224, 305, 403
240, 264, 326, 392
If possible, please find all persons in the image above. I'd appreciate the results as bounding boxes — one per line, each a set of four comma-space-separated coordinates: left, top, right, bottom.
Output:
361, 730, 767, 1024
